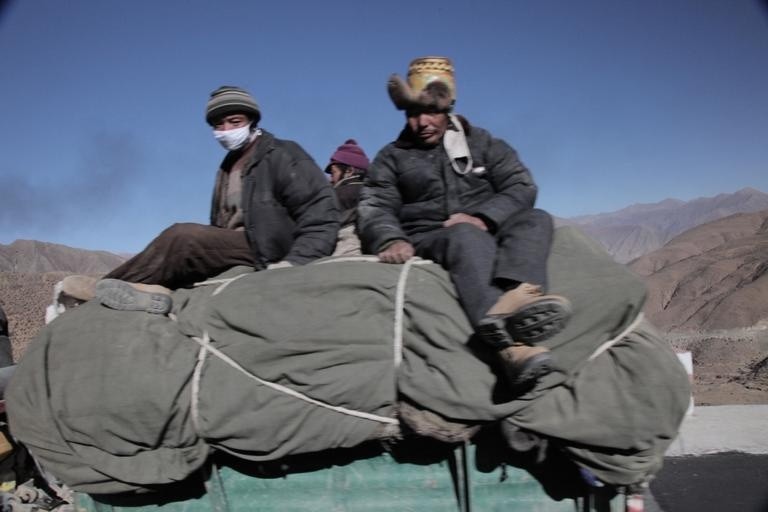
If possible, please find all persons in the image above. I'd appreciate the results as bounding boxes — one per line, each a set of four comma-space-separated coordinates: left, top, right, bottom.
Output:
324, 140, 372, 228
60, 85, 339, 313
324, 56, 572, 385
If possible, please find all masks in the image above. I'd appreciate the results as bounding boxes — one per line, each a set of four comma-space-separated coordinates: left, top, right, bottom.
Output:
442, 113, 472, 175
212, 122, 253, 151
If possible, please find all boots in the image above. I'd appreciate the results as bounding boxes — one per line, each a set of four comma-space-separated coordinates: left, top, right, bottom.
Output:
477, 282, 573, 385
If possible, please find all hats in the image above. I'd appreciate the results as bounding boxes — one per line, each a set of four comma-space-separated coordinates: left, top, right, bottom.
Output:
324, 139, 369, 173
205, 85, 261, 127
388, 56, 457, 110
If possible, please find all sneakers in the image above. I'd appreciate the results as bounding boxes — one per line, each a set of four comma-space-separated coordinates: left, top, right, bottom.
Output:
61, 274, 172, 315
